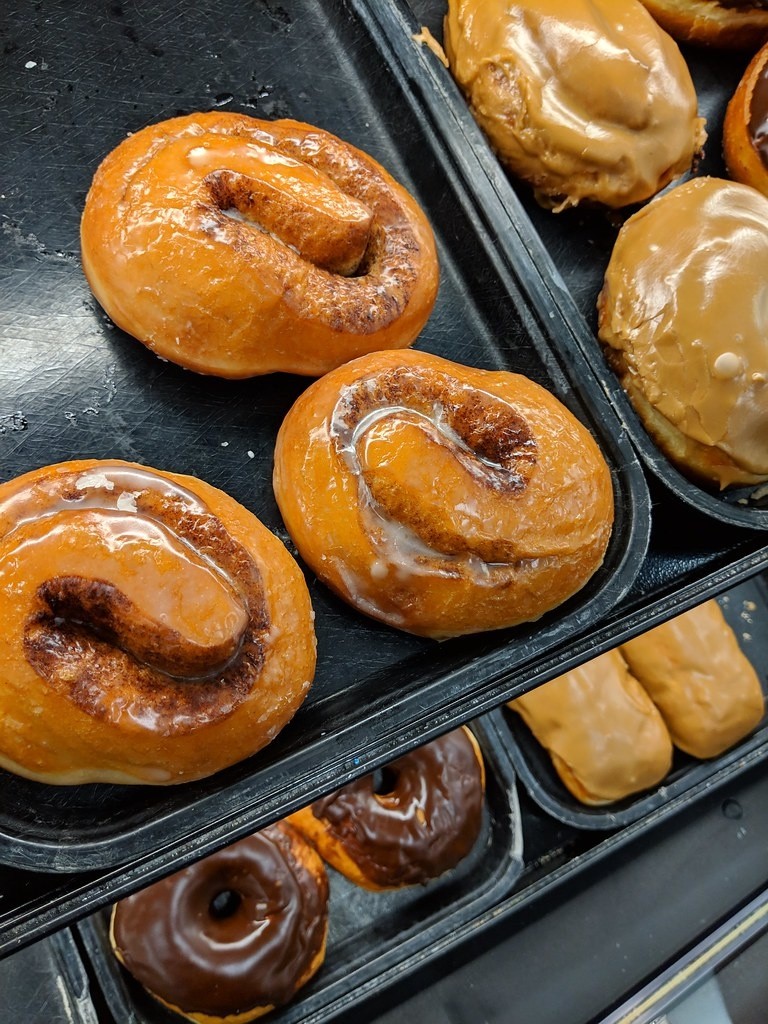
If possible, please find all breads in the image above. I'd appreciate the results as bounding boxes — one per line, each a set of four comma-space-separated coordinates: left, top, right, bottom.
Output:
0, 0, 766, 782
512, 599, 766, 808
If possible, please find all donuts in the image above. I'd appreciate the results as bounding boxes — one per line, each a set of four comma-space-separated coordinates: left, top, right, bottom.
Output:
106, 818, 333, 1023
289, 723, 486, 891
